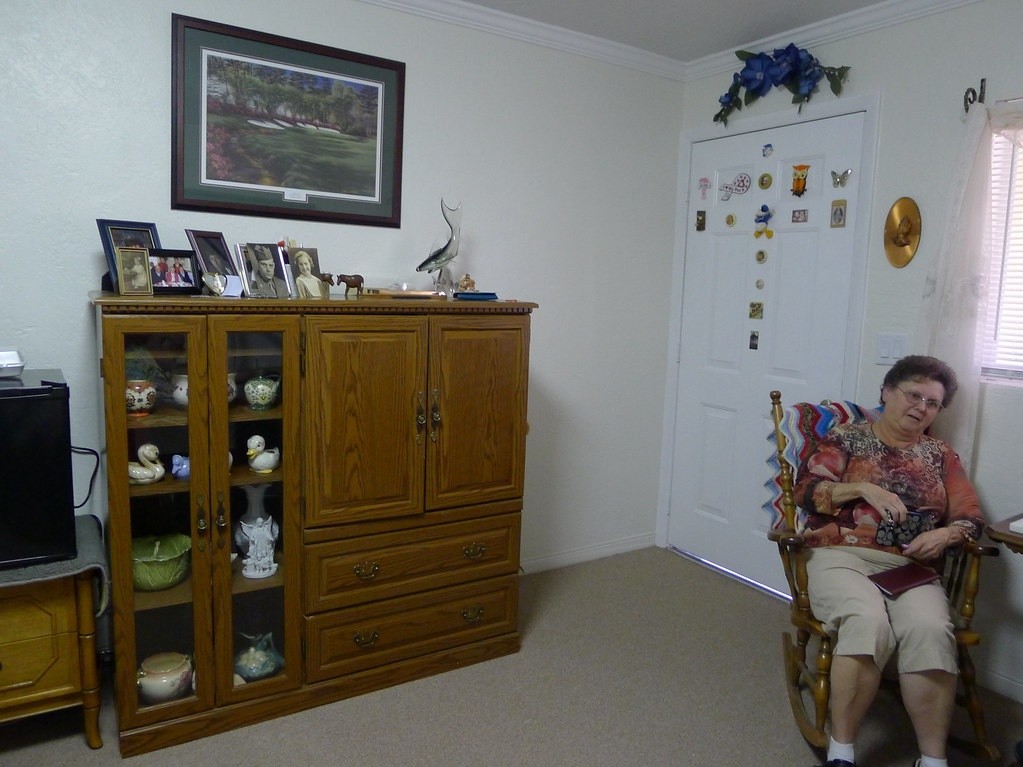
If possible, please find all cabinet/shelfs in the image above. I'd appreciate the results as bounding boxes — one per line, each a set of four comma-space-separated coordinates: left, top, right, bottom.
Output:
300, 505, 525, 687
0, 515, 109, 752
96, 302, 307, 734
303, 301, 538, 529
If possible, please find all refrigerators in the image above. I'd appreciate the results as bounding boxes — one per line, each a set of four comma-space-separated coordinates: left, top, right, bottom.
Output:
0, 370, 78, 572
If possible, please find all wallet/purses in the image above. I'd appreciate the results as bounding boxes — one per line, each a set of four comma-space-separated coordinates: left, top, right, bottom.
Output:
877, 503, 934, 546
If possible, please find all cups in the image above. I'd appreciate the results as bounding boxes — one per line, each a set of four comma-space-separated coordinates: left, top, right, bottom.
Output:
171, 374, 189, 409
227, 372, 237, 403
125, 380, 157, 417
244, 373, 281, 411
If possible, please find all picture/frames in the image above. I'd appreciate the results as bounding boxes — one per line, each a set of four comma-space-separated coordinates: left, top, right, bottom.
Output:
168, 11, 407, 231
287, 246, 328, 299
115, 245, 154, 297
234, 242, 293, 298
185, 228, 239, 276
147, 247, 203, 295
96, 218, 164, 293
246, 241, 290, 300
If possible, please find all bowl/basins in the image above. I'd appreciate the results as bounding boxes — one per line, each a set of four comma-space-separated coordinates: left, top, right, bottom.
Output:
135, 652, 193, 705
131, 534, 192, 591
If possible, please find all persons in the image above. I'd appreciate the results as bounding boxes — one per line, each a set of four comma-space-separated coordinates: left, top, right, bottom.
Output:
173, 256, 183, 275
158, 256, 168, 276
294, 251, 324, 299
130, 257, 147, 291
151, 265, 165, 285
795, 355, 986, 767
207, 250, 234, 275
177, 264, 192, 285
166, 267, 182, 287
253, 245, 289, 298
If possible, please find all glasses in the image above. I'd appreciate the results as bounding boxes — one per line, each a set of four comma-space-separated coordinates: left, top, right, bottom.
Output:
889, 380, 945, 412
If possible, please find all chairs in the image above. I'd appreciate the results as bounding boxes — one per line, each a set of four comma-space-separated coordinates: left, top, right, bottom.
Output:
760, 388, 1002, 767
